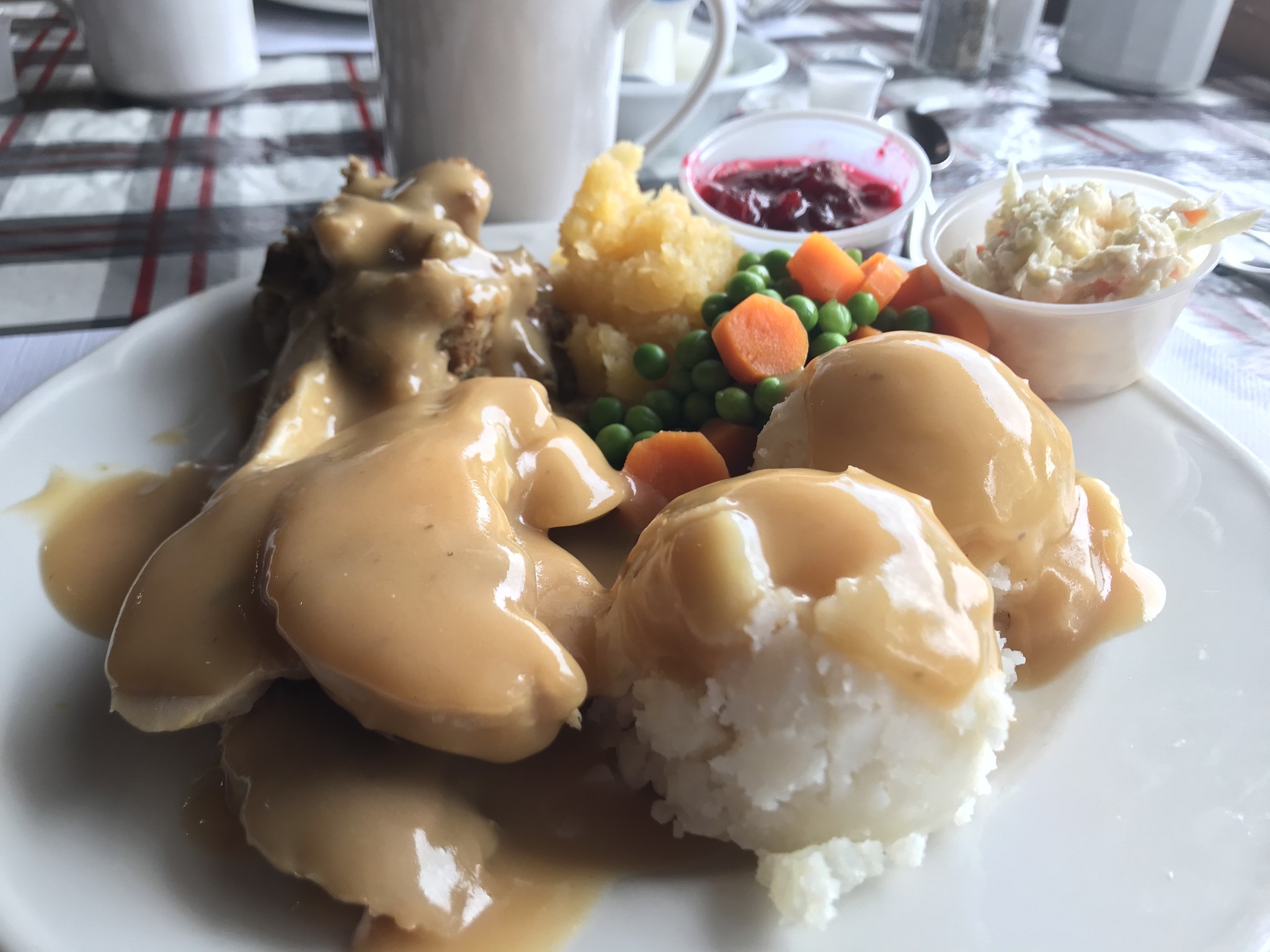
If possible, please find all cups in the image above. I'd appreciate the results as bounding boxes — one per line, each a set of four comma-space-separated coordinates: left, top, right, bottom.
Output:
368, 0, 731, 220
1060, 2, 1235, 91
996, 0, 1043, 76
924, 165, 1218, 404
71, 1, 259, 107
680, 109, 927, 256
910, 0, 995, 79
799, 50, 895, 123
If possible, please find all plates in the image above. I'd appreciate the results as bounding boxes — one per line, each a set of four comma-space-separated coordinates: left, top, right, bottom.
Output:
2, 209, 1270, 952
617, 20, 788, 153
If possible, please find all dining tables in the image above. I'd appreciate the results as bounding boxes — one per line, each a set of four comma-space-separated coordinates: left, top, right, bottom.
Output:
0, 0, 1270, 952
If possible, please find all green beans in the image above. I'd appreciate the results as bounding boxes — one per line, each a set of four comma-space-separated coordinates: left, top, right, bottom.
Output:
576, 246, 931, 467
